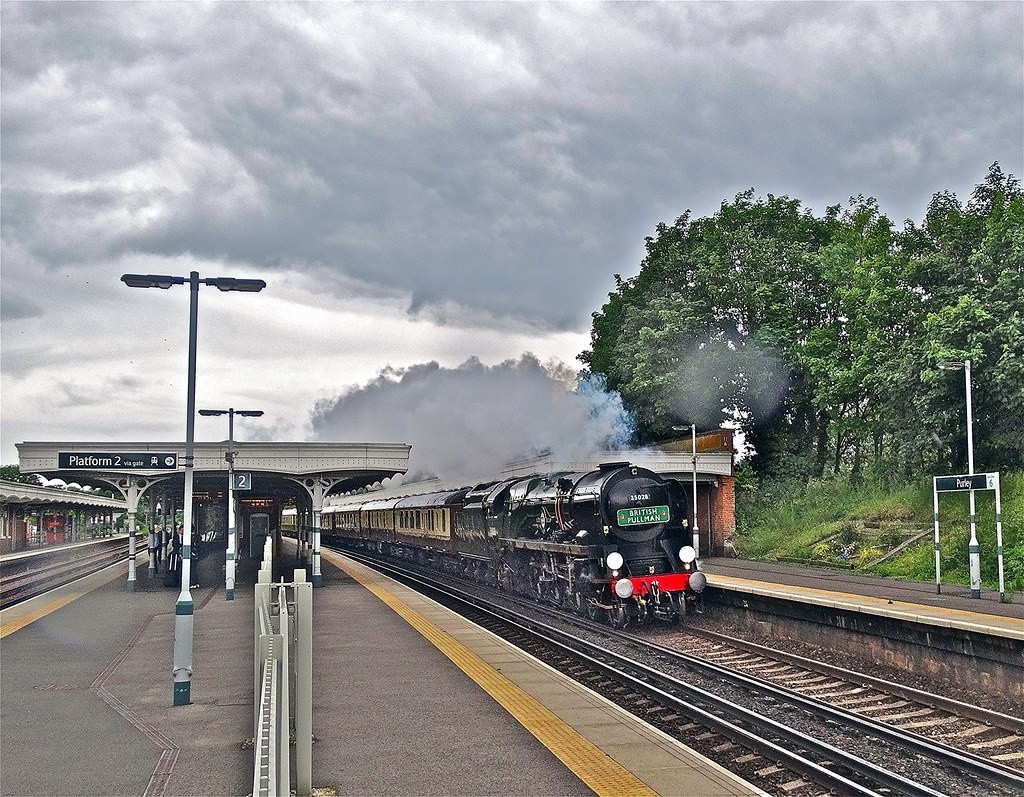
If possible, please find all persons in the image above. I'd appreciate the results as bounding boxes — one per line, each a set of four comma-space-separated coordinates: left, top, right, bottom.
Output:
156, 525, 169, 561
172, 523, 200, 588
146, 526, 159, 563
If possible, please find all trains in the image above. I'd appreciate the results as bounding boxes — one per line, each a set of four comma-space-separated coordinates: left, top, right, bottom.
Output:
281, 460, 707, 630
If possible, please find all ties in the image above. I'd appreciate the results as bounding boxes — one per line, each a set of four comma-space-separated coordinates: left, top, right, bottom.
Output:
181, 536, 183, 542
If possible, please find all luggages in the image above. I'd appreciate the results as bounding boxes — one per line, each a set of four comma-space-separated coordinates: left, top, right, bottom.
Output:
163, 554, 179, 586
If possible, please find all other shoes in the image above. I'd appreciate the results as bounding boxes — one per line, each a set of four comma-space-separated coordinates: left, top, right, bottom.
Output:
196, 584, 199, 588
190, 585, 196, 589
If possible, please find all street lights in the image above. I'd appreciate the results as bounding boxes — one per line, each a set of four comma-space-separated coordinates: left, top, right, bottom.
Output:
672, 424, 703, 572
935, 358, 982, 599
197, 409, 265, 602
119, 271, 267, 705
